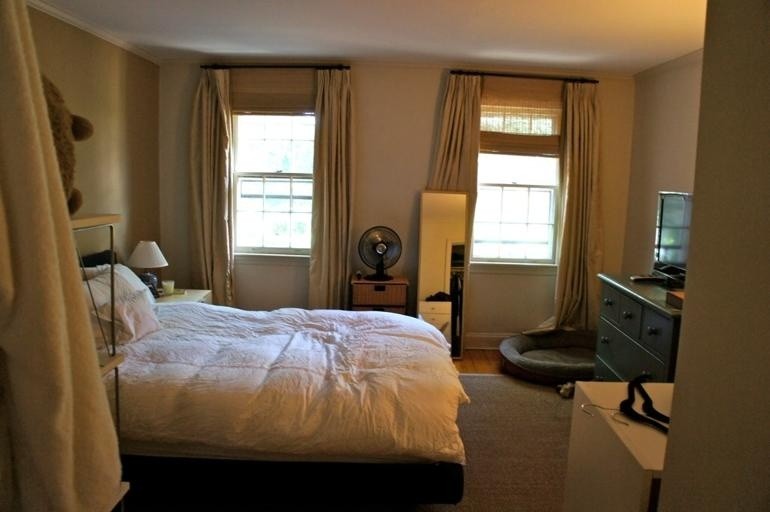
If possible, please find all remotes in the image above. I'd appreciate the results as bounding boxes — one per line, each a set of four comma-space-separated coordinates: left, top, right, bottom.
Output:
630, 274, 666, 283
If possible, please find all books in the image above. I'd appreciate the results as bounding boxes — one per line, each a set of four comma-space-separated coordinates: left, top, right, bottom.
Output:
666, 291, 683, 309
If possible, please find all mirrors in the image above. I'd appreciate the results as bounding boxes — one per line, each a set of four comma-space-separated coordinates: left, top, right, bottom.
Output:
416, 191, 468, 362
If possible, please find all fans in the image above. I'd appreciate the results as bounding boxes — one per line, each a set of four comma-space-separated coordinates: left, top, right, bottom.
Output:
357, 224, 403, 282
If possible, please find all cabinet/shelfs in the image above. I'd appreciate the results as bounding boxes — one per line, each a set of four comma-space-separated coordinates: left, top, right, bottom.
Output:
348, 273, 410, 317
74, 210, 131, 511
589, 270, 689, 384
417, 299, 453, 346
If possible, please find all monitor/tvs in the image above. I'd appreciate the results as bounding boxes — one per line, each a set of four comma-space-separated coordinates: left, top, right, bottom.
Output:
654, 191, 693, 284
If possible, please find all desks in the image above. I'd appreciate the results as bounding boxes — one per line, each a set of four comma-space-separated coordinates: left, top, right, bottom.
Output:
560, 378, 680, 509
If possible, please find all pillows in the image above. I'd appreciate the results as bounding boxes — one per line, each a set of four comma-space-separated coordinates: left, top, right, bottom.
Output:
75, 264, 162, 348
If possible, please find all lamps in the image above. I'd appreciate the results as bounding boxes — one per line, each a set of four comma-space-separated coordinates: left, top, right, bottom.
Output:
127, 238, 170, 298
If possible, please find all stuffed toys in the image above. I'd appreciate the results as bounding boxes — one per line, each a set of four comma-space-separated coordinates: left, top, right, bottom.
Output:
39, 73, 93, 214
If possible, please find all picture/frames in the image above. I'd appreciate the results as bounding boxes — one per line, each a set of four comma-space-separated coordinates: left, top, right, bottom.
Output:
146, 283, 158, 298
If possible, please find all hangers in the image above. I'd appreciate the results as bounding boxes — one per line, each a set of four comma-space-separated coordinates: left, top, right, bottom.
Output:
580, 379, 669, 438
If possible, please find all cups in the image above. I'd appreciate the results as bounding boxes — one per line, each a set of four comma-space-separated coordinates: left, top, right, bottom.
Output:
161, 280, 175, 295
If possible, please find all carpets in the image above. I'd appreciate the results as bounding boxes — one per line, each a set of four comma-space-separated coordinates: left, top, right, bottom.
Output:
109, 371, 579, 511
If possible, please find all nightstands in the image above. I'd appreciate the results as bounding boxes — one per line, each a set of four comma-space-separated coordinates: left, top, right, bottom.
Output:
151, 288, 214, 306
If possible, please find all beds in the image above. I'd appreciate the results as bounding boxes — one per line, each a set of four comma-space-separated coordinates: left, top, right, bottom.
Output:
72, 249, 468, 505
